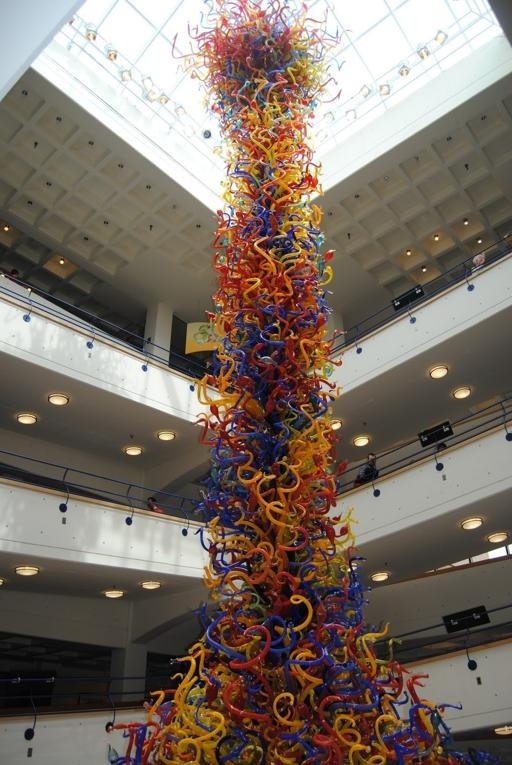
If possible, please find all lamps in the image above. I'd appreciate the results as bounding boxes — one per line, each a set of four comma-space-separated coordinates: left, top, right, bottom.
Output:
17, 413, 37, 423
48, 392, 69, 405
339, 562, 358, 573
158, 430, 174, 440
0, 566, 160, 598
494, 726, 512, 735
454, 387, 471, 398
487, 532, 508, 543
460, 517, 484, 530
125, 446, 142, 454
330, 420, 342, 430
429, 365, 448, 378
354, 436, 369, 446
369, 570, 392, 582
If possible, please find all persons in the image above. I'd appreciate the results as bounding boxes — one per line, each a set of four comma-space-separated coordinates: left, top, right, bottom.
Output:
146, 496, 164, 513
436, 442, 447, 452
471, 252, 485, 273
7, 268, 20, 283
351, 453, 379, 490
